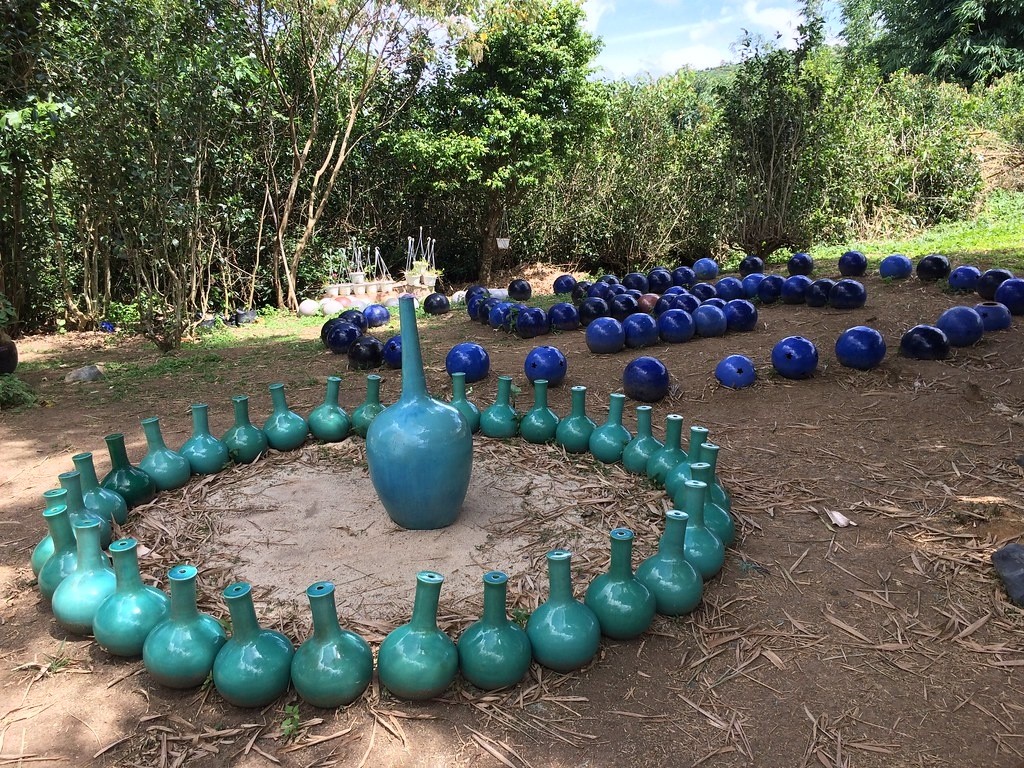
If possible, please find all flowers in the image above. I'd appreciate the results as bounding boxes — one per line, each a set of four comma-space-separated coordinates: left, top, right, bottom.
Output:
325, 274, 342, 286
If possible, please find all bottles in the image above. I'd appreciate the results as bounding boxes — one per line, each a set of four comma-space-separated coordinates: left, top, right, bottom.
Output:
30, 295, 735, 708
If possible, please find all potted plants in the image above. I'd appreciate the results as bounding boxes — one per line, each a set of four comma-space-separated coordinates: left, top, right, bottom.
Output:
195, 133, 269, 329
337, 260, 396, 296
0, 292, 25, 376
399, 259, 446, 287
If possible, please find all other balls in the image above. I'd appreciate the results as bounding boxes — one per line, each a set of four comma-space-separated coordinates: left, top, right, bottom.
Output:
320, 250, 1024, 405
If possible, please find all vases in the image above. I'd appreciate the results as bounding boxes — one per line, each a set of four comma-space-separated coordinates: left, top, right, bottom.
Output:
326, 285, 340, 296
